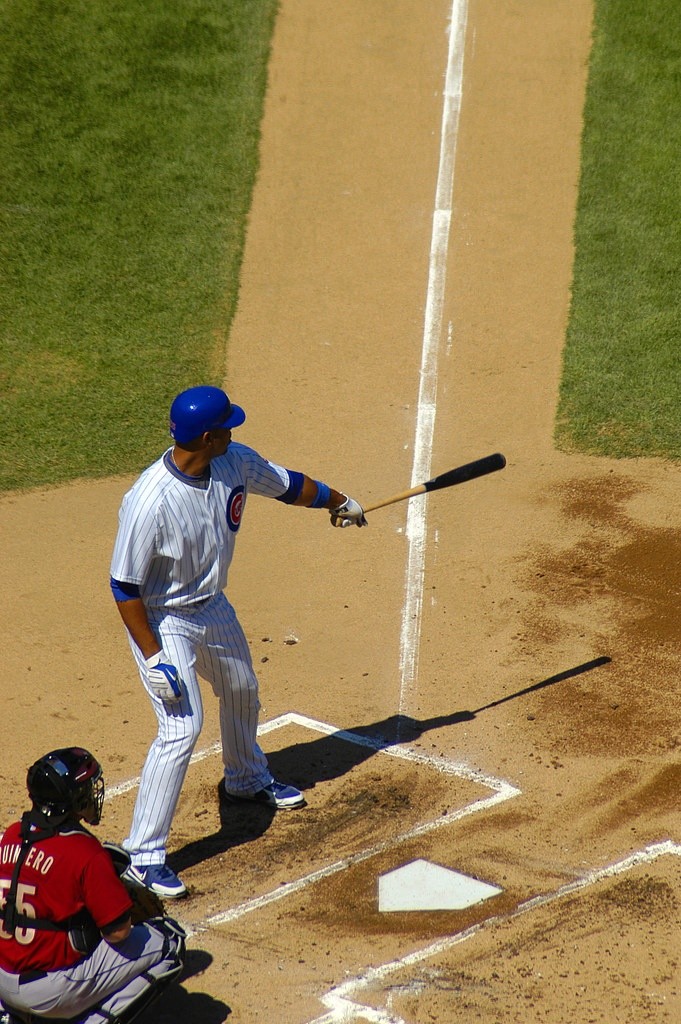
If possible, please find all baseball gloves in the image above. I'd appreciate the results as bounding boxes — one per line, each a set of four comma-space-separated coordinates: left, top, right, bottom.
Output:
122, 879, 166, 927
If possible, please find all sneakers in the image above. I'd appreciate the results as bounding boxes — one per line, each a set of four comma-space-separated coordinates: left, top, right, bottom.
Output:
224, 781, 305, 808
124, 864, 187, 900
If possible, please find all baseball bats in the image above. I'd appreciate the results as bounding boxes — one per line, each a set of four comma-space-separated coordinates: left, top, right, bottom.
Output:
330, 451, 506, 526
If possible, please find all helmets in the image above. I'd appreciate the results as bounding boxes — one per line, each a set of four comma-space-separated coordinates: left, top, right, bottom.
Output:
27, 747, 102, 815
169, 385, 246, 442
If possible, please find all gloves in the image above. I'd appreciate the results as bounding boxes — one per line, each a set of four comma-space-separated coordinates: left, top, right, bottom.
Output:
146, 648, 187, 705
328, 492, 369, 528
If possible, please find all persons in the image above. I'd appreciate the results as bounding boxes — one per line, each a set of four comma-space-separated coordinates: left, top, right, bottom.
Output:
0, 747, 185, 1024
110, 386, 365, 900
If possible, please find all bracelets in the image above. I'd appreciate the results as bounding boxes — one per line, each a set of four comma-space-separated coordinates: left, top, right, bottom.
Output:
308, 481, 330, 508
